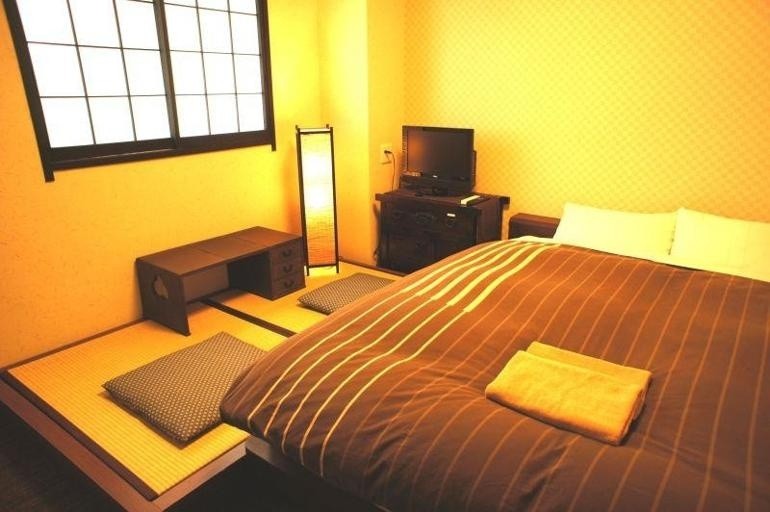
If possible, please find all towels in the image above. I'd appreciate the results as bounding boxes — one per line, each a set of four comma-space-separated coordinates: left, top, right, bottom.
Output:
527, 340, 652, 420
485, 350, 642, 444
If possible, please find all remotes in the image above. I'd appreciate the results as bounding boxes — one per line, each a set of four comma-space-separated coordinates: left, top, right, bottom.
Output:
461, 195, 481, 204
466, 197, 491, 206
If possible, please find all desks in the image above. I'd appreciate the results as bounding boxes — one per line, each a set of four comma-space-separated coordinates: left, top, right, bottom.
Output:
136, 226, 306, 335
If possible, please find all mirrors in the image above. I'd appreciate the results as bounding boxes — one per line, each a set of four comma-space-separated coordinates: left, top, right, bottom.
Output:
295, 125, 339, 267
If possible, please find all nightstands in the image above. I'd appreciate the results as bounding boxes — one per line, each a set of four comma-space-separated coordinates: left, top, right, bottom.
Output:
508, 213, 560, 240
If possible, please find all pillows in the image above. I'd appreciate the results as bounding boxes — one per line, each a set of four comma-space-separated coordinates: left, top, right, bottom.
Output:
553, 202, 676, 264
102, 330, 272, 445
669, 206, 770, 285
297, 274, 396, 313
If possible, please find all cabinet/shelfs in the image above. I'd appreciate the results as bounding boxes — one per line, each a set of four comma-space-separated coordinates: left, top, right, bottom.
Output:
374, 188, 510, 274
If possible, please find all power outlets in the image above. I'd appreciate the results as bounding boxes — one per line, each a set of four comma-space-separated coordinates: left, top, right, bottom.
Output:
381, 143, 393, 163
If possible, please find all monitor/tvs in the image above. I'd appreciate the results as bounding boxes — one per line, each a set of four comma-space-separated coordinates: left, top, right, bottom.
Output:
398, 125, 476, 195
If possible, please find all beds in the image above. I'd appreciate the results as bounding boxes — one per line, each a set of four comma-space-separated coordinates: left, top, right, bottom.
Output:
218, 213, 770, 512
